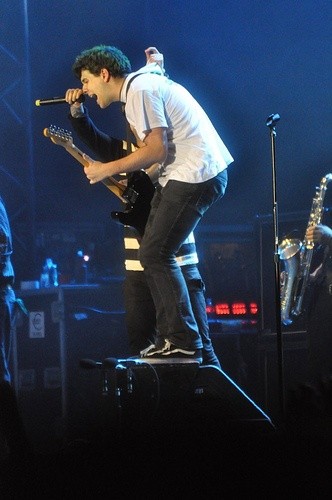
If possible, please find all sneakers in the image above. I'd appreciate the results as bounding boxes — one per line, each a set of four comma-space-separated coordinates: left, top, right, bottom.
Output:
128, 340, 203, 365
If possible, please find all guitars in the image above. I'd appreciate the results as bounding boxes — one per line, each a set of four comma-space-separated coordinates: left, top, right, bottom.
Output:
44, 125, 156, 246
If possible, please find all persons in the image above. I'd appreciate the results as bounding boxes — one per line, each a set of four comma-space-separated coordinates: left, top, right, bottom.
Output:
0, 199, 17, 463
74, 45, 235, 362
65, 47, 222, 369
286, 225, 332, 468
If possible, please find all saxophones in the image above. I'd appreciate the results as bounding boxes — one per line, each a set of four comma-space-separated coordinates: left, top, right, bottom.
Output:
276, 173, 332, 325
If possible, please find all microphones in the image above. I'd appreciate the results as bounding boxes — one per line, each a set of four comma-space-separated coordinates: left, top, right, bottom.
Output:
103, 357, 129, 374
80, 358, 102, 368
36, 94, 86, 106
